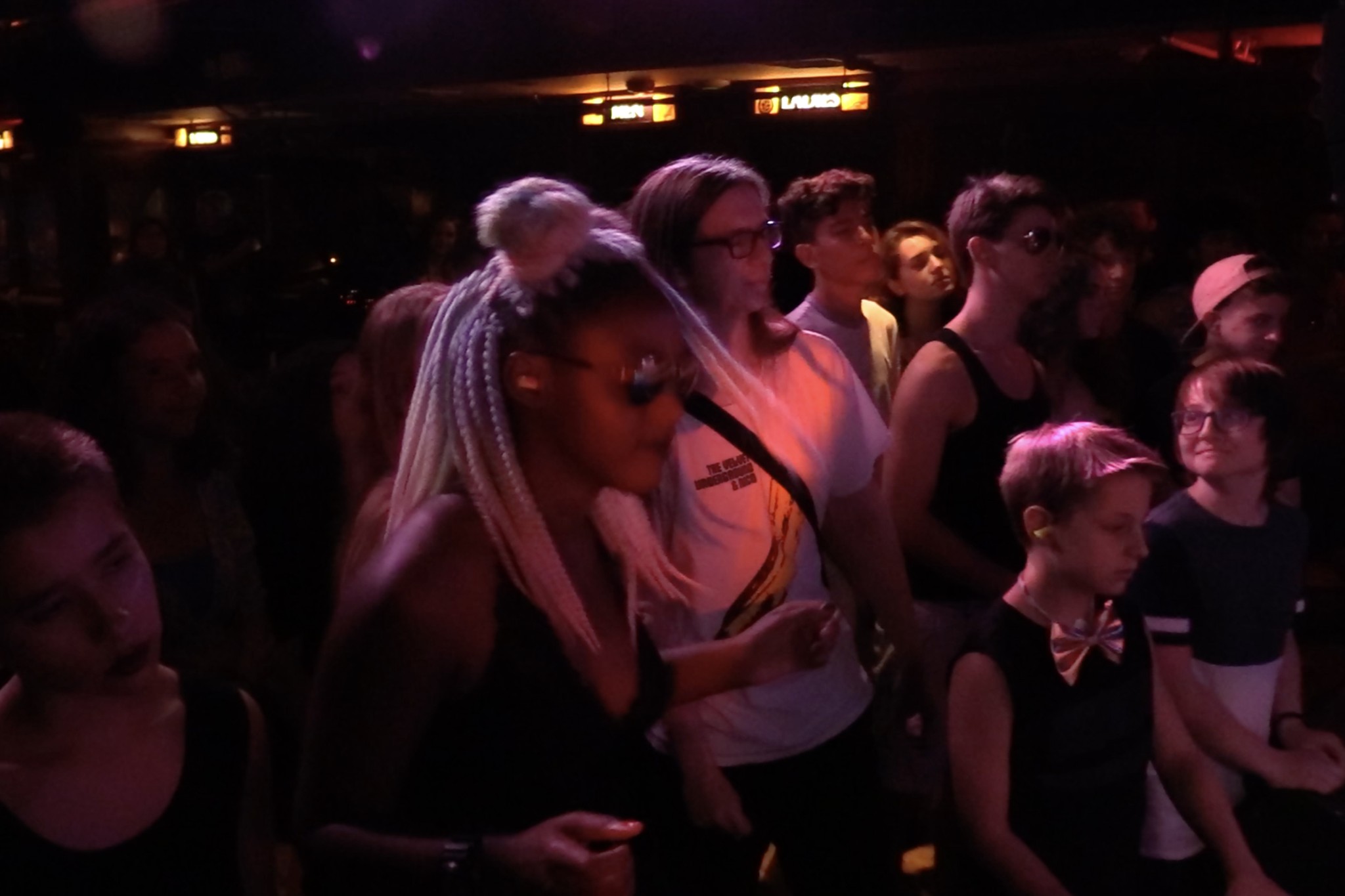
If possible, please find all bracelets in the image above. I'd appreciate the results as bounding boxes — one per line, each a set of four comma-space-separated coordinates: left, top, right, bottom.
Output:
443, 840, 470, 877
1274, 711, 1307, 743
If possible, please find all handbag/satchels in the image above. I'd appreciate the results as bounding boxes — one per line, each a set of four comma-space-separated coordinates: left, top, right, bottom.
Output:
862, 626, 921, 725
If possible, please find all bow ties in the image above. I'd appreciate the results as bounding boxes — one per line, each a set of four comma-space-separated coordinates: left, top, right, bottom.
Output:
1052, 599, 1125, 682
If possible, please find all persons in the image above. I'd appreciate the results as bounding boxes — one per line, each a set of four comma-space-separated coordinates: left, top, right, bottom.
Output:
0, 148, 1345, 896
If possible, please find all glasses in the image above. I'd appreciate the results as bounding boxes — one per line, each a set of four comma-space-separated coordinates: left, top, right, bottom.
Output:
530, 347, 701, 404
1174, 402, 1250, 439
698, 220, 784, 258
1006, 227, 1053, 256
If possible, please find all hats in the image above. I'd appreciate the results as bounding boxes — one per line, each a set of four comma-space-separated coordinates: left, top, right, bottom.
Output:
1178, 252, 1281, 348
